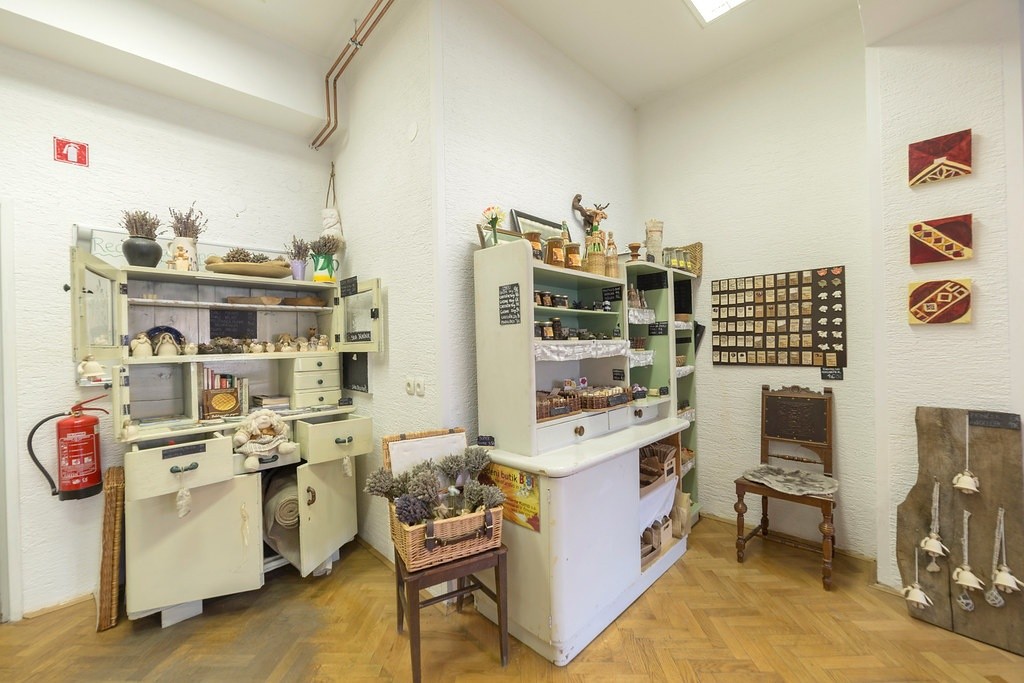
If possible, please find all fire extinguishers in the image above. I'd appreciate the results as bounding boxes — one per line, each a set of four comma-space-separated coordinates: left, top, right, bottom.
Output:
27, 392, 110, 501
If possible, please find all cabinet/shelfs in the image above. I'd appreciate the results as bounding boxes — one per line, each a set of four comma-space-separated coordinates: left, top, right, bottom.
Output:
473, 239, 704, 666
70, 247, 382, 629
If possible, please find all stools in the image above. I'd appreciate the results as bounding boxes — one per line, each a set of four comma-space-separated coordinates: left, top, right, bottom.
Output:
394, 541, 509, 683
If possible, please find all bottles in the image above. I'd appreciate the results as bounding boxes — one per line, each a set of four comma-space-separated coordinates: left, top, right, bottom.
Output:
589, 231, 604, 252
606, 231, 618, 255
561, 221, 569, 243
582, 236, 592, 258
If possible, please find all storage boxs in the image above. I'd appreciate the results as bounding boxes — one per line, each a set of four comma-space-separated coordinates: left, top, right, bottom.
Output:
640, 457, 677, 500
382, 426, 504, 573
640, 514, 673, 567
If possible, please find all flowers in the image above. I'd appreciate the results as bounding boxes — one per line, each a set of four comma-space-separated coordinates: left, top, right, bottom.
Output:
118, 209, 169, 239
167, 200, 208, 238
309, 234, 341, 255
284, 235, 310, 264
362, 446, 507, 527
480, 206, 508, 246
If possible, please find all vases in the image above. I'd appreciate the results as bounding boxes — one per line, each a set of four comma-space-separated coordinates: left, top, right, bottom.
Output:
289, 259, 310, 281
164, 236, 199, 272
121, 235, 162, 268
309, 253, 339, 284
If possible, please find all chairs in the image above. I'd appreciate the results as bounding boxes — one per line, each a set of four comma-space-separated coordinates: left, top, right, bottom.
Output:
733, 384, 837, 592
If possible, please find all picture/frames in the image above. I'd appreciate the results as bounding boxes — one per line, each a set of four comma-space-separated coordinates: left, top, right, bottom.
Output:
511, 209, 573, 263
477, 224, 524, 249
